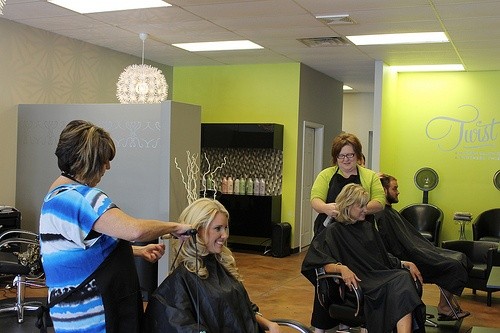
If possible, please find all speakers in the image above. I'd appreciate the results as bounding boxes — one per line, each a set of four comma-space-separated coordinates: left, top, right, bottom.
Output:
271, 223, 291, 258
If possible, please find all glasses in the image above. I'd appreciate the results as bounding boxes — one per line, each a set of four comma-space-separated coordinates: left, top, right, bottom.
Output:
336, 152, 356, 159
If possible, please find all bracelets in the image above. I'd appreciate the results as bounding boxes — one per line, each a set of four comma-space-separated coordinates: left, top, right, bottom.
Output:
335, 262, 342, 274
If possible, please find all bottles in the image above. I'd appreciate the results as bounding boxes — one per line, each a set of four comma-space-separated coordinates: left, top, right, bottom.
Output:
201, 175, 265, 196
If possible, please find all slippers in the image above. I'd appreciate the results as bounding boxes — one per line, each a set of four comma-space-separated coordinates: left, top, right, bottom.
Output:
454, 305, 471, 316
437, 309, 467, 321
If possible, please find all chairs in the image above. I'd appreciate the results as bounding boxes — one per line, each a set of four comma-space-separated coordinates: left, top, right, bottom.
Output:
251, 313, 316, 333
396, 201, 445, 256
442, 206, 500, 309
308, 215, 429, 333
0, 206, 51, 327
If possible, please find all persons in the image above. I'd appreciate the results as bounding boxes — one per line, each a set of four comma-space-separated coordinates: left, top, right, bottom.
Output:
310, 133, 385, 333
39, 120, 196, 333
358, 153, 366, 168
374, 173, 474, 321
145, 197, 281, 333
300, 182, 427, 333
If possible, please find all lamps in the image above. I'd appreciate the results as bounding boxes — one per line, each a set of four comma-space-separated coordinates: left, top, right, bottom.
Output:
109, 33, 171, 107
